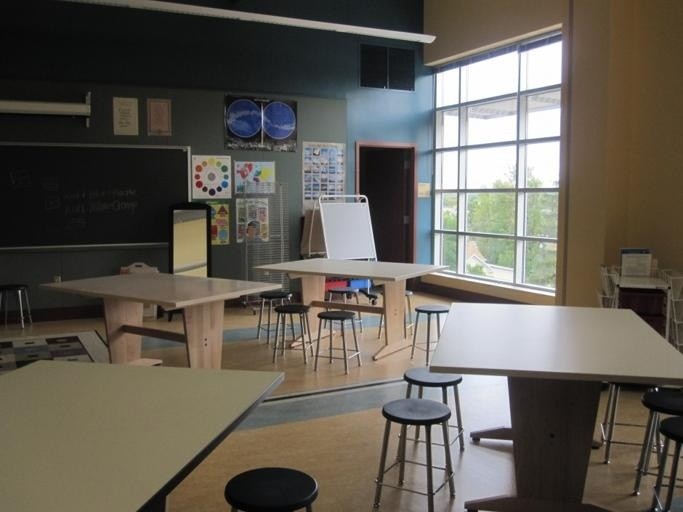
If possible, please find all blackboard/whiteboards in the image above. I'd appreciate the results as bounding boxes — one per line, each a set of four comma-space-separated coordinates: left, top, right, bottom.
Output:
0, 141, 192, 251
319, 202, 376, 261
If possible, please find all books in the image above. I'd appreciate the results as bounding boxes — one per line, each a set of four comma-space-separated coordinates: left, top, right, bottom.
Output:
617, 246, 652, 276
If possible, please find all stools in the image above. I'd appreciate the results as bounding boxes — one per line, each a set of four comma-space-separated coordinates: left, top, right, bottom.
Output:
222, 467, 317, 511
652, 416, 683, 512
600, 380, 661, 467
373, 397, 456, 511
631, 387, 683, 496
399, 367, 464, 453
256, 286, 412, 374
407, 306, 448, 365
1, 284, 32, 329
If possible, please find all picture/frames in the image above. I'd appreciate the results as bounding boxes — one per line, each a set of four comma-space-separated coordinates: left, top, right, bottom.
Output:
146, 98, 173, 138
113, 96, 139, 136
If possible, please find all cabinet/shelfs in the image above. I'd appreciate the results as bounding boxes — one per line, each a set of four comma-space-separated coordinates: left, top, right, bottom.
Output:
596, 268, 617, 309
663, 268, 683, 352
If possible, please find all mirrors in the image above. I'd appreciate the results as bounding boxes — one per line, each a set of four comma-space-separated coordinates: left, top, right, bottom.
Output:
168, 202, 212, 322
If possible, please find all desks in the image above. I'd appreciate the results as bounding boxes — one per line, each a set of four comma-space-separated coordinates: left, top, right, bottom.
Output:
38, 274, 282, 368
252, 257, 447, 363
0, 360, 285, 512
613, 277, 671, 342
429, 302, 683, 512
